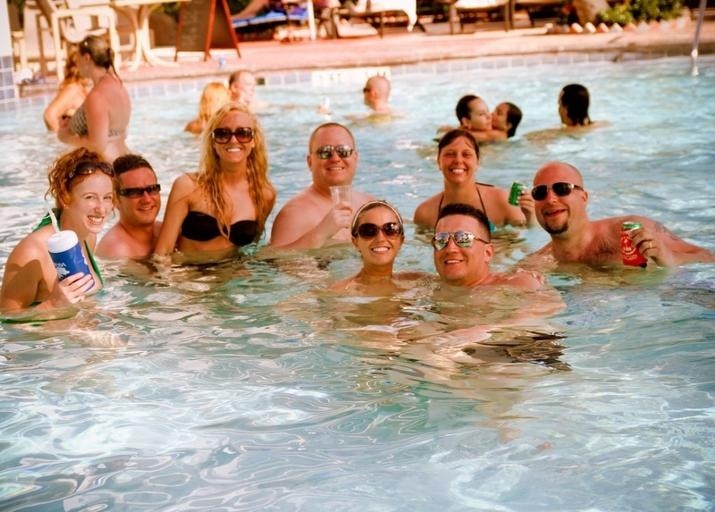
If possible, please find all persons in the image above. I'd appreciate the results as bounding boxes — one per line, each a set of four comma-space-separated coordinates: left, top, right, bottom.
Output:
277, 201, 437, 344
56, 35, 132, 166
414, 129, 536, 264
184, 83, 228, 133
228, 70, 314, 113
270, 123, 381, 280
391, 204, 570, 445
502, 162, 714, 309
95, 154, 170, 283
45, 49, 89, 130
153, 103, 276, 283
0, 154, 113, 326
525, 84, 608, 145
436, 102, 522, 148
414, 94, 492, 164
314, 76, 402, 125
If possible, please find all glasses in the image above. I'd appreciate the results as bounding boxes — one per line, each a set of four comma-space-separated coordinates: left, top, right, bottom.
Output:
531, 183, 584, 201
354, 222, 403, 242
67, 162, 115, 184
116, 185, 160, 199
316, 145, 354, 159
432, 230, 488, 252
211, 127, 255, 144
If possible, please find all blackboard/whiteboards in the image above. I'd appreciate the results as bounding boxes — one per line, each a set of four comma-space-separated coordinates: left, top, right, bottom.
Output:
176, 0, 239, 51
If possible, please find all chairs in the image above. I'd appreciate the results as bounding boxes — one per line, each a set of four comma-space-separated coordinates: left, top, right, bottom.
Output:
232, 0, 518, 48
13, 1, 176, 80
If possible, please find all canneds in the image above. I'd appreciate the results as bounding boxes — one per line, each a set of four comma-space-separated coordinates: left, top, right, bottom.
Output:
619, 221, 647, 266
508, 180, 525, 206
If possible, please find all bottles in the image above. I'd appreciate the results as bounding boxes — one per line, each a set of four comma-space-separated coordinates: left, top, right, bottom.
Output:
48, 230, 95, 294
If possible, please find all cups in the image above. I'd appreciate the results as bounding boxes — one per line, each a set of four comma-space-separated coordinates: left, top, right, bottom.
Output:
329, 185, 353, 207
